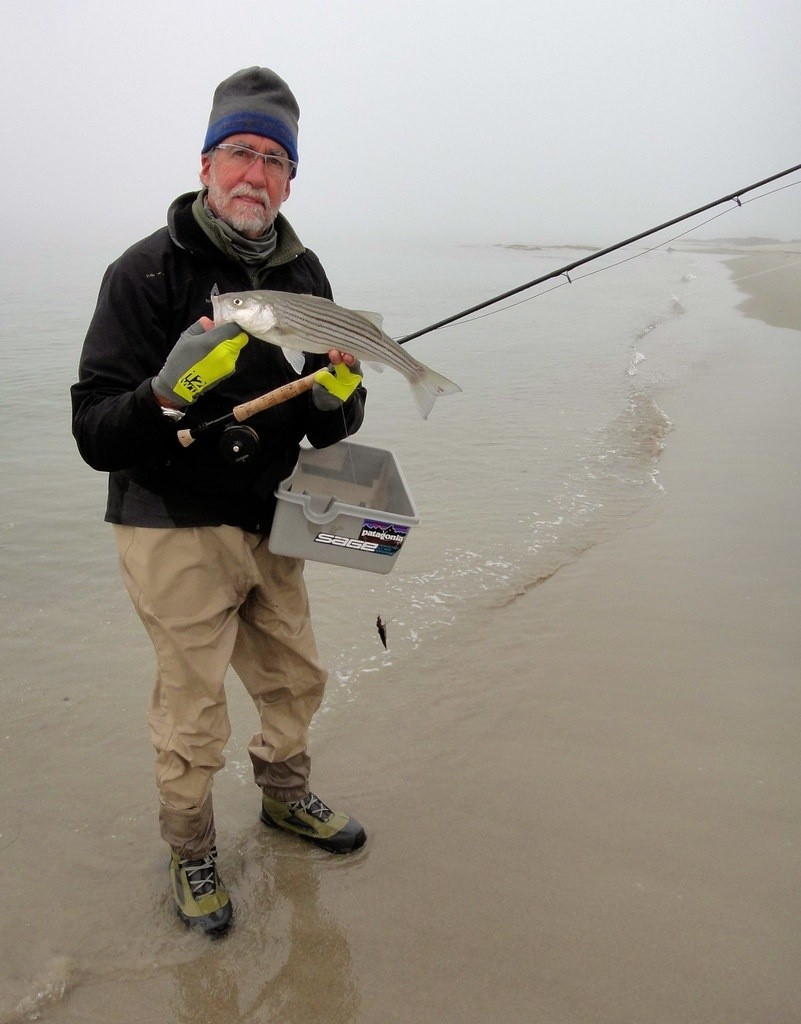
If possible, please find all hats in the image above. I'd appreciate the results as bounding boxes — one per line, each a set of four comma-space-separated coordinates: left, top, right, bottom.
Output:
202, 66, 301, 181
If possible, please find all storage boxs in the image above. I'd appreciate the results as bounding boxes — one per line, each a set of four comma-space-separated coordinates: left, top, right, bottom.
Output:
267, 434, 420, 574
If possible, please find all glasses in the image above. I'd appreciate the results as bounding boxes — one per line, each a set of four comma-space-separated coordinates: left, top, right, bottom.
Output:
212, 143, 298, 180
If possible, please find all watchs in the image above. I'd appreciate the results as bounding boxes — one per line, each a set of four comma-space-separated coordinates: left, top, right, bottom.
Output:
160, 406, 185, 422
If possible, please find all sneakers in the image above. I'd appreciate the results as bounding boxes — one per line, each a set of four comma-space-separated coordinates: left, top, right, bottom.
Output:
256, 794, 366, 855
167, 849, 234, 937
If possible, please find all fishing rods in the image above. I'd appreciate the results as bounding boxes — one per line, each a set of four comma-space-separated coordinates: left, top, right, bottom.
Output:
176, 162, 800, 443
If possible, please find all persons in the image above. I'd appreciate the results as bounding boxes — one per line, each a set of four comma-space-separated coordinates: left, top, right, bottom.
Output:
73, 62, 367, 942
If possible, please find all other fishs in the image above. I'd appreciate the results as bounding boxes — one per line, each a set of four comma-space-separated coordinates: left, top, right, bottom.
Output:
376, 615, 388, 651
210, 288, 463, 420
